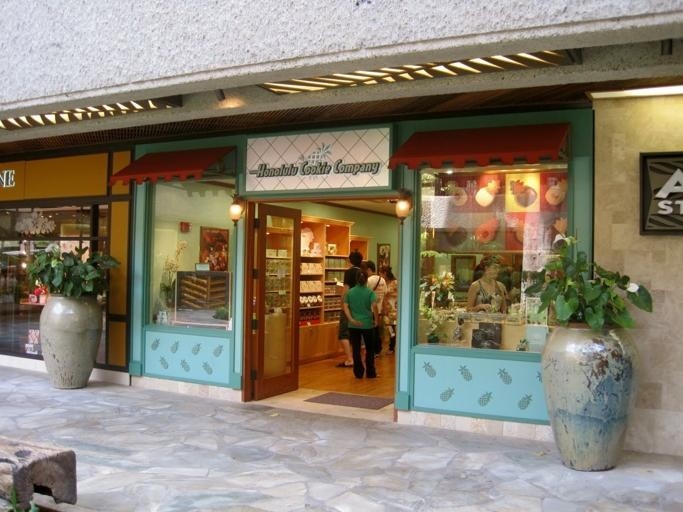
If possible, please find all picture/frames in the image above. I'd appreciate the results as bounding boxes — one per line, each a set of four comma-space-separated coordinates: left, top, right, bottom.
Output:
198, 225, 229, 271
376, 243, 391, 277
449, 254, 475, 292
638, 149, 682, 236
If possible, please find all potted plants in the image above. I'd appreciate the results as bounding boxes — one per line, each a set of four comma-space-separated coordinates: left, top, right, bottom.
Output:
31, 240, 115, 392
523, 235, 651, 473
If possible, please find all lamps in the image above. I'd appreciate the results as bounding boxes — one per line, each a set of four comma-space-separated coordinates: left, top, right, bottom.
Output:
386, 186, 413, 223
228, 191, 248, 227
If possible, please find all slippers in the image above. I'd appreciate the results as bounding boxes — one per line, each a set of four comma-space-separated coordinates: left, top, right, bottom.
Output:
335, 361, 353, 367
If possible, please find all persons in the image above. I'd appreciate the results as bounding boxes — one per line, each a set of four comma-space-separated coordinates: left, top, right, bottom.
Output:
336, 250, 397, 379
467, 254, 509, 314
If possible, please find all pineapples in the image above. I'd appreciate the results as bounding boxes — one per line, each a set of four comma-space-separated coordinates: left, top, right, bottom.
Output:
441, 181, 468, 206
507, 218, 537, 244
511, 180, 537, 208
475, 179, 501, 209
546, 179, 567, 205
308, 142, 329, 166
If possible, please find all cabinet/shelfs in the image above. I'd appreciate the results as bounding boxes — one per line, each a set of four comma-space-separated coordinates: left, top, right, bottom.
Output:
252, 213, 375, 374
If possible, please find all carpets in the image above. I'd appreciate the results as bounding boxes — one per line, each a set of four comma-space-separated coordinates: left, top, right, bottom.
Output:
303, 392, 395, 411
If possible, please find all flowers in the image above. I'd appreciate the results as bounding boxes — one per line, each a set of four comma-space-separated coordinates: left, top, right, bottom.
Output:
419, 264, 455, 334
